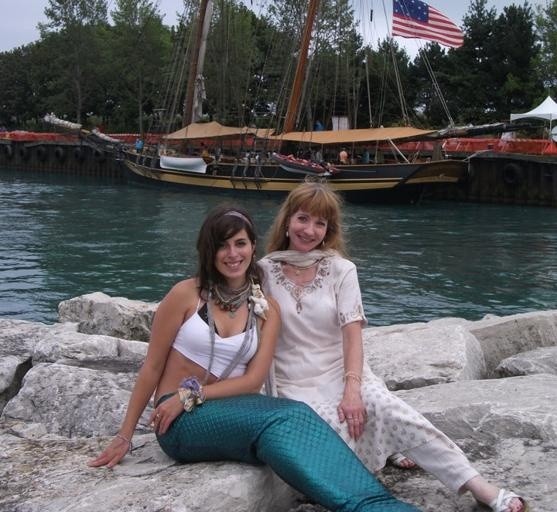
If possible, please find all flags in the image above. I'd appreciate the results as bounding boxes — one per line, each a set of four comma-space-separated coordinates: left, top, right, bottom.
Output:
388, 1, 465, 52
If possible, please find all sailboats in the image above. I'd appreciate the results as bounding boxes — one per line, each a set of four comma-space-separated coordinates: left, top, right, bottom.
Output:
45, 0, 547, 202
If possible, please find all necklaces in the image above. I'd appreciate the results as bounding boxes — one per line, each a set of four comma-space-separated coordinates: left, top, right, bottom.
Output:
206, 278, 252, 319
289, 265, 310, 276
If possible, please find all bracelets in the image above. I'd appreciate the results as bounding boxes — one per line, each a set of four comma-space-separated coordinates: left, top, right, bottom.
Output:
341, 369, 363, 387
177, 375, 206, 414
115, 431, 133, 454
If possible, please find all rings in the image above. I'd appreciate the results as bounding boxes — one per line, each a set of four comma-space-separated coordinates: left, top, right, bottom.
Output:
155, 412, 160, 418
346, 415, 358, 424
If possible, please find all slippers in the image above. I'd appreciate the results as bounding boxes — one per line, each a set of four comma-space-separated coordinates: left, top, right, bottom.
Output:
477, 487, 527, 512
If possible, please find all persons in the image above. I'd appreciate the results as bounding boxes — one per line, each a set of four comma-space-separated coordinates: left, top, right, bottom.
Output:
131, 137, 389, 166
82, 200, 424, 512
252, 172, 532, 512
